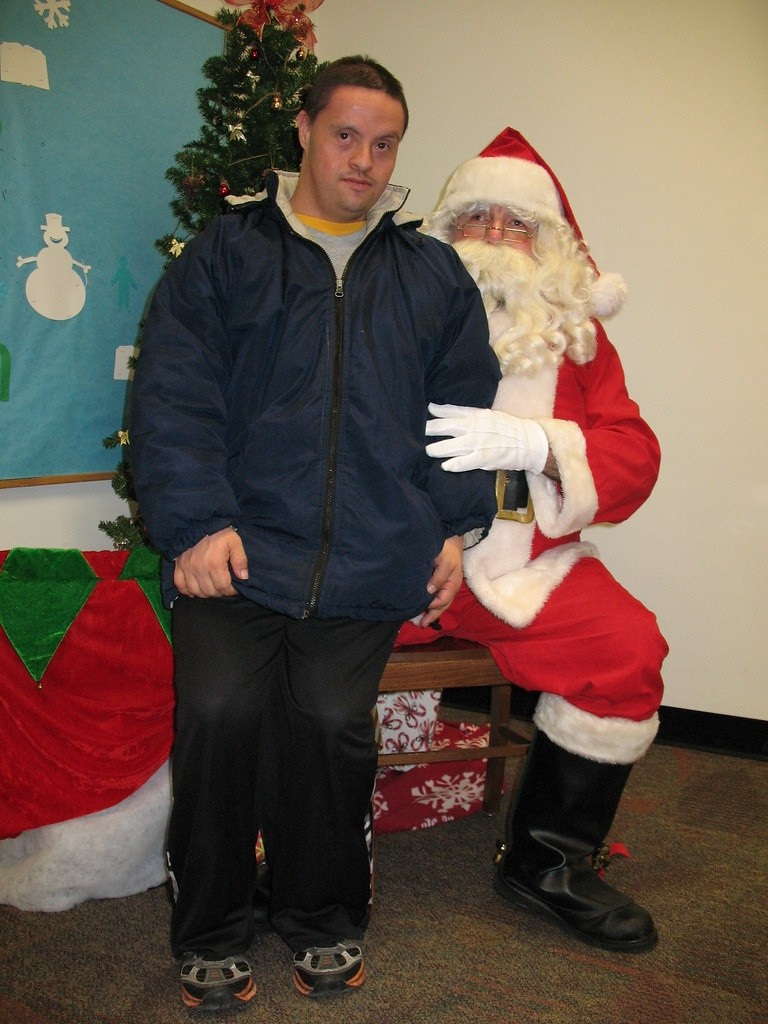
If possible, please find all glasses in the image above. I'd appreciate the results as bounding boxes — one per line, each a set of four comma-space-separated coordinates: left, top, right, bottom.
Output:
449, 222, 536, 243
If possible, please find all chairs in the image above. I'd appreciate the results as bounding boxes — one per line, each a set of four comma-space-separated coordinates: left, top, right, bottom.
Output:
367, 637, 537, 858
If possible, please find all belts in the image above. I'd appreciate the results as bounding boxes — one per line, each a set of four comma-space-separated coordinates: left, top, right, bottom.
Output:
490, 469, 535, 524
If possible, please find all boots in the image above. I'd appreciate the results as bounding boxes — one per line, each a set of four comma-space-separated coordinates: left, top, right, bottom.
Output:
493, 728, 661, 955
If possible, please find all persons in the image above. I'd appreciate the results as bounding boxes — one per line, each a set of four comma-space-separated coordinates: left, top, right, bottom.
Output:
125, 49, 506, 1016
381, 119, 670, 960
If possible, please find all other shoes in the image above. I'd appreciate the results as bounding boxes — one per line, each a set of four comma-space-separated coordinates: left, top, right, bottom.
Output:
291, 939, 368, 999
179, 948, 256, 1011
